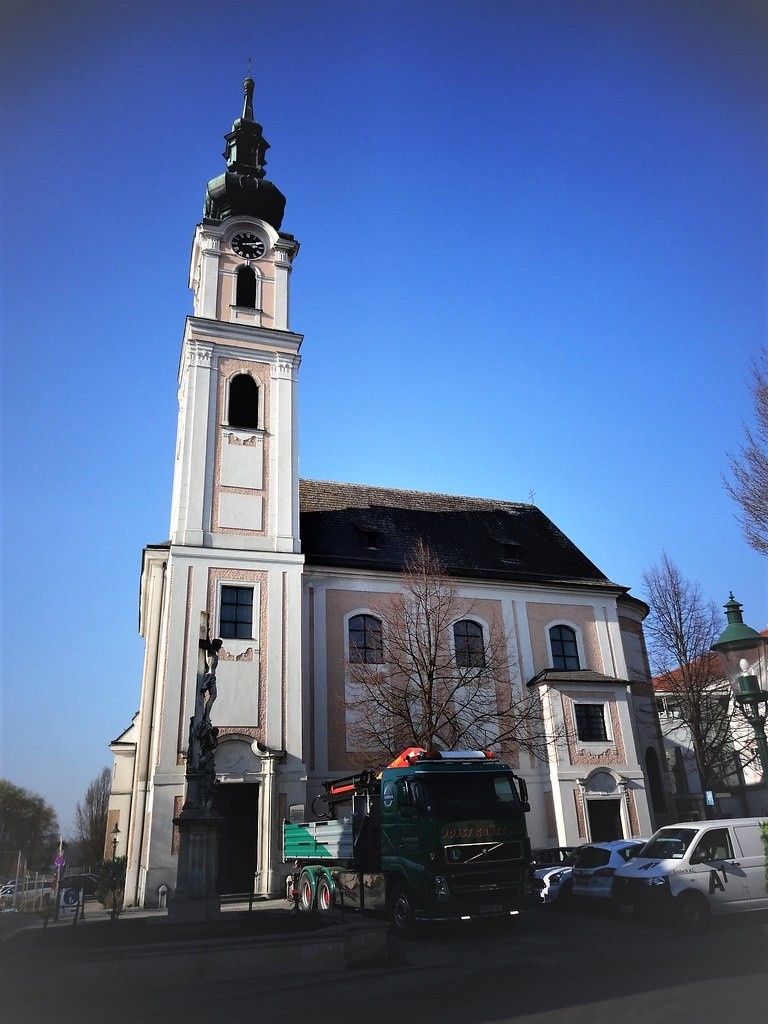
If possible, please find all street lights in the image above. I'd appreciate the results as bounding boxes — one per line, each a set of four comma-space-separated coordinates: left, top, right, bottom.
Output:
711, 588, 768, 787
110, 821, 122, 862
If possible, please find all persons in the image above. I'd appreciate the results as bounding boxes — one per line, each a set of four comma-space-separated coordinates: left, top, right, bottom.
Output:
198, 621, 224, 725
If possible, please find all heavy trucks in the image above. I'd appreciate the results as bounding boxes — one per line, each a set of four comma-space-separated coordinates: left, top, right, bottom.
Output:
282, 740, 539, 936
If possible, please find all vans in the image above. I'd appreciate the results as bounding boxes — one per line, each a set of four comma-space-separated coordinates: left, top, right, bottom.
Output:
609, 816, 768, 930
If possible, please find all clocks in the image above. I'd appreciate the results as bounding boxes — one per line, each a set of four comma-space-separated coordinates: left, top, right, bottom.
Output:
229, 230, 267, 261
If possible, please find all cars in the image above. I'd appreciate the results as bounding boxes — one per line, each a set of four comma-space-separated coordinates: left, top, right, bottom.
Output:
0, 872, 102, 907
531, 835, 683, 900
